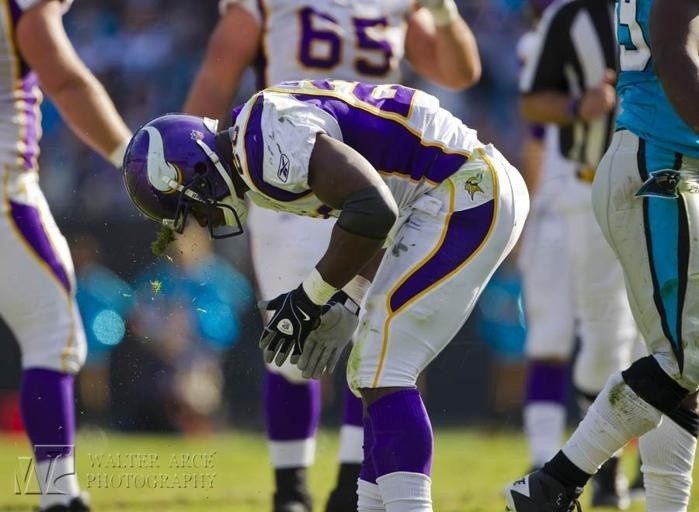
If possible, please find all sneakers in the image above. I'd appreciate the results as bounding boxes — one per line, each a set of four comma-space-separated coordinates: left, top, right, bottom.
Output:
326, 490, 357, 512
592, 470, 620, 510
504, 470, 584, 512
274, 490, 311, 512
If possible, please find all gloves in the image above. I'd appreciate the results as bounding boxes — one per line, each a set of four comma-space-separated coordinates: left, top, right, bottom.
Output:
298, 290, 362, 380
258, 281, 327, 366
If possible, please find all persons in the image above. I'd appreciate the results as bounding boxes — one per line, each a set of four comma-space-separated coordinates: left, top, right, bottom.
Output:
1, 0, 699, 512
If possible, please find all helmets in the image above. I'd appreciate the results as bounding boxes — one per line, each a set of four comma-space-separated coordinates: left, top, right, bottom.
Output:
122, 113, 248, 240
